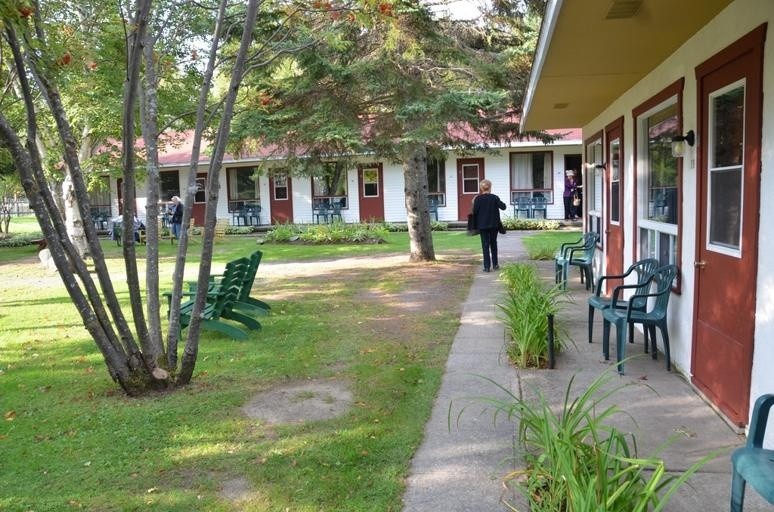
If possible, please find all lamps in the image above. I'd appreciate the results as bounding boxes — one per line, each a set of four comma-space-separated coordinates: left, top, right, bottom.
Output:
671, 129, 695, 158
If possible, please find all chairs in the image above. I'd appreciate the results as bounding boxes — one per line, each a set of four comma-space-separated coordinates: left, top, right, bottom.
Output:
555, 232, 597, 294
587, 259, 659, 353
514, 197, 547, 219
331, 202, 343, 224
601, 265, 679, 376
317, 203, 330, 225
163, 252, 271, 343
233, 205, 261, 226
428, 199, 438, 222
138, 217, 227, 246
730, 394, 771, 510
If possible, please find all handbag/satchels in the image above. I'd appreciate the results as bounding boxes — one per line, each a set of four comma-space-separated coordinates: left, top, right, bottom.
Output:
573, 195, 581, 206
467, 213, 480, 235
498, 223, 506, 234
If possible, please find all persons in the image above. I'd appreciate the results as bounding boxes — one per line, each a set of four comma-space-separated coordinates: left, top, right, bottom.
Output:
472, 179, 507, 273
568, 168, 580, 219
170, 196, 185, 240
563, 170, 577, 220
